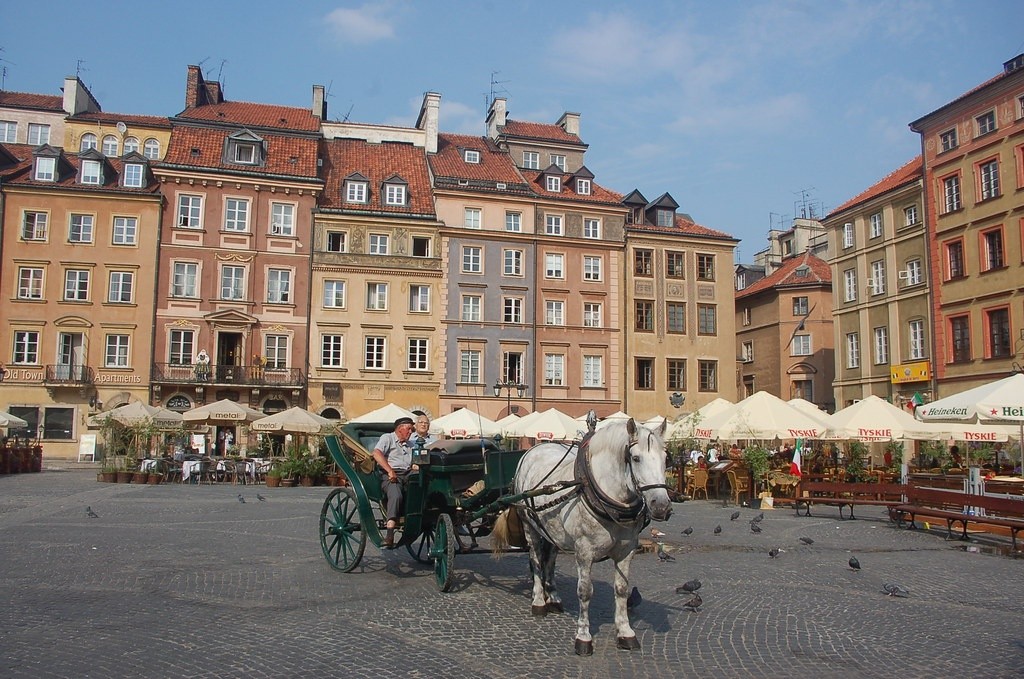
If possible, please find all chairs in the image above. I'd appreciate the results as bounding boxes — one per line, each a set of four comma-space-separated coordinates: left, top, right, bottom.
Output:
727, 470, 750, 505
139, 453, 272, 486
688, 468, 709, 502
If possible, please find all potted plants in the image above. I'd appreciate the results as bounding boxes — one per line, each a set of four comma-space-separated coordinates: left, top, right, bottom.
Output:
93, 417, 162, 484
265, 437, 342, 487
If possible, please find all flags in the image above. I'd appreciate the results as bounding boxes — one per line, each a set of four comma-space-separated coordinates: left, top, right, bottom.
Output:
789, 439, 802, 486
907, 391, 924, 410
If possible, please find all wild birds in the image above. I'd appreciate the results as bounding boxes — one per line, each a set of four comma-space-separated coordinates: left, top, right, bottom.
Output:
627, 587, 642, 614
238, 494, 246, 503
849, 556, 861, 570
683, 594, 703, 613
680, 526, 693, 536
799, 537, 814, 545
749, 512, 764, 533
882, 581, 908, 597
256, 493, 266, 502
768, 547, 780, 557
675, 578, 701, 593
650, 525, 666, 539
85, 505, 98, 519
730, 511, 739, 520
656, 542, 676, 563
713, 524, 722, 535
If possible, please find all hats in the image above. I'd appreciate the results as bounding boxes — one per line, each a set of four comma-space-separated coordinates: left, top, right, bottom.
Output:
394, 418, 415, 426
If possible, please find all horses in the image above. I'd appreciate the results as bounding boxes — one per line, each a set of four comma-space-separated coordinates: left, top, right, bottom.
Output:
490, 417, 673, 657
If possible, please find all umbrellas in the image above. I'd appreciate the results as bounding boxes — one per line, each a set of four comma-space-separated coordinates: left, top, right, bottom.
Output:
87, 399, 212, 457
0, 410, 28, 428
183, 398, 270, 457
342, 373, 1024, 479
249, 406, 343, 458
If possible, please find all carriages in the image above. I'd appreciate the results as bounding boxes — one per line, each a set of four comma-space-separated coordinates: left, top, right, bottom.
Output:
319, 408, 672, 657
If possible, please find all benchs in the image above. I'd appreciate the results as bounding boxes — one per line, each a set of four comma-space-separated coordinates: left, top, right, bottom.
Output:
426, 439, 511, 472
792, 471, 903, 521
892, 476, 1024, 555
356, 437, 408, 500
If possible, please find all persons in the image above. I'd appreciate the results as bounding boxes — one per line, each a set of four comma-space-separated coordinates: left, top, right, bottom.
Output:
408, 415, 438, 449
884, 449, 892, 467
729, 444, 742, 458
372, 417, 419, 546
951, 446, 962, 468
811, 455, 823, 482
690, 445, 704, 464
707, 444, 721, 462
696, 456, 706, 468
994, 443, 1009, 469
210, 427, 233, 458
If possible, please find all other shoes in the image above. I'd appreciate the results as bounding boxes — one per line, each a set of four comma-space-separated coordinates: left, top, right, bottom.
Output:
385, 532, 394, 545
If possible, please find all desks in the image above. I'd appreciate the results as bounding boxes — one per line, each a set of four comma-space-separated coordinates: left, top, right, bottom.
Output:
141, 460, 157, 473
182, 460, 201, 485
212, 460, 236, 479
245, 457, 270, 481
